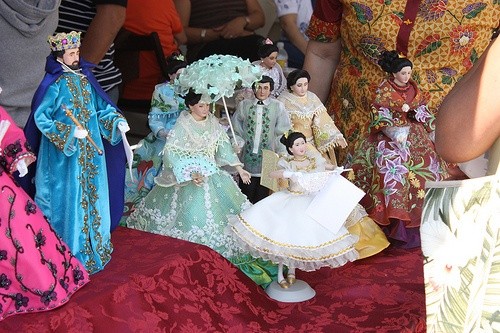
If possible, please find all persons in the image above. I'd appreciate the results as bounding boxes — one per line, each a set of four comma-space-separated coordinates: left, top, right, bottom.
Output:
1, 0, 500, 208
0, 30, 452, 318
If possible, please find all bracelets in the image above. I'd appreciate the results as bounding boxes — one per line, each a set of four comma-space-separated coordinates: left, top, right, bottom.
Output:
243, 16, 250, 28
200, 28, 206, 38
